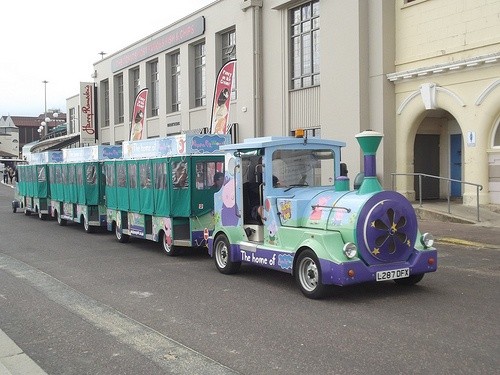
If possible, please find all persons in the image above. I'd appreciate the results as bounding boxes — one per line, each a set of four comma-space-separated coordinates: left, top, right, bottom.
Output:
210, 171, 225, 193
3, 164, 15, 184
247, 174, 279, 227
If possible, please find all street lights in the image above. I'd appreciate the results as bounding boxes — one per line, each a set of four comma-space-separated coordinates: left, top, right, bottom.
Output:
37, 117, 50, 138
53, 113, 58, 126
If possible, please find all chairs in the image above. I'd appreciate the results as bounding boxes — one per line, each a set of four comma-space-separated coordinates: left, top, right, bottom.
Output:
243, 182, 263, 222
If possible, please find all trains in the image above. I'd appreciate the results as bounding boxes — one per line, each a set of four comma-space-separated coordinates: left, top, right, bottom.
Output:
13, 130, 438, 298
